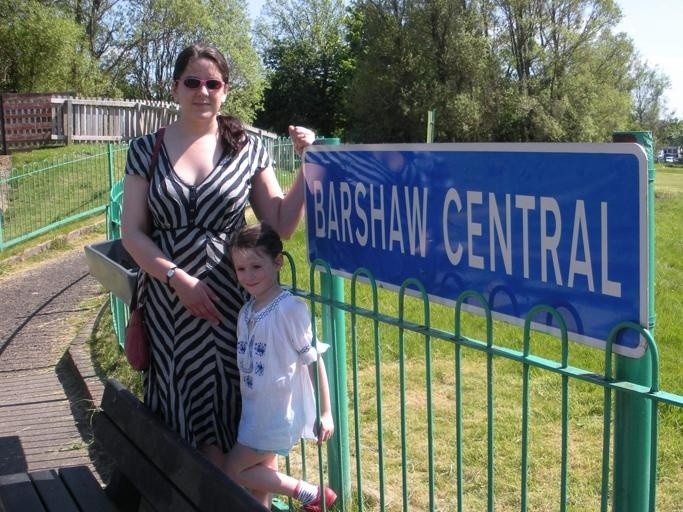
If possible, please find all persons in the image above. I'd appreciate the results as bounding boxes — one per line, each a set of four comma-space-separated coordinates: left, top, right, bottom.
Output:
222, 221, 341, 510
119, 43, 316, 487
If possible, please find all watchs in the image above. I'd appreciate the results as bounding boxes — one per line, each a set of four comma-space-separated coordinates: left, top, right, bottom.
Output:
165, 267, 179, 288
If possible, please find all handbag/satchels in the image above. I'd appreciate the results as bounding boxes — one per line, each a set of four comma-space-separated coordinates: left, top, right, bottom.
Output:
125, 310, 149, 370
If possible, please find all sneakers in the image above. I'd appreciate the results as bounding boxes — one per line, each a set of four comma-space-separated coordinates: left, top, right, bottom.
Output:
303, 486, 337, 512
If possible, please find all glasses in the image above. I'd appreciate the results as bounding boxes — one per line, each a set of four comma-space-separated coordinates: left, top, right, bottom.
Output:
176, 79, 225, 89
